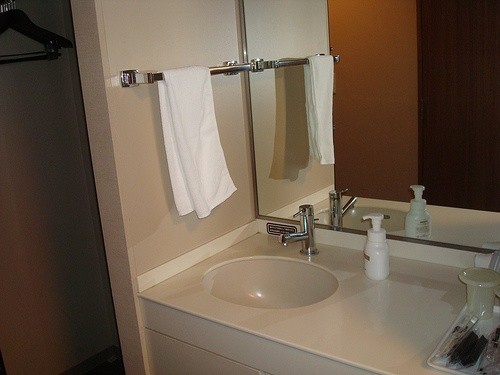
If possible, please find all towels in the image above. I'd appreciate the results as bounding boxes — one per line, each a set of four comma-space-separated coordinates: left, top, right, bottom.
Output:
303, 54, 335, 165
158, 64, 238, 220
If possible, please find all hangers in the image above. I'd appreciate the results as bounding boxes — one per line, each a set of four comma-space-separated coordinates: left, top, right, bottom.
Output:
0, 0, 73, 64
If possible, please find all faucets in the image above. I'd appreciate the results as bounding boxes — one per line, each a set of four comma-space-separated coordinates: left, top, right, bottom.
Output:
342, 196, 358, 216
279, 231, 308, 247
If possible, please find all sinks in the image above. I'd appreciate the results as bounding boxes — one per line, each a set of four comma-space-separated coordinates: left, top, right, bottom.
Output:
203, 258, 339, 309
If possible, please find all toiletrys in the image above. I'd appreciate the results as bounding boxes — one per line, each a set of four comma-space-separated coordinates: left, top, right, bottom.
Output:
362, 213, 390, 280
405, 184, 432, 239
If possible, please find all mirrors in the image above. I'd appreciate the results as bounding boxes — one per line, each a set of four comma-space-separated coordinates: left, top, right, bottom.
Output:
234, 0, 500, 256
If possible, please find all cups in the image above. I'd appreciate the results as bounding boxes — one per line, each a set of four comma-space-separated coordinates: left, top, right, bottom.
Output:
459, 267, 500, 319
